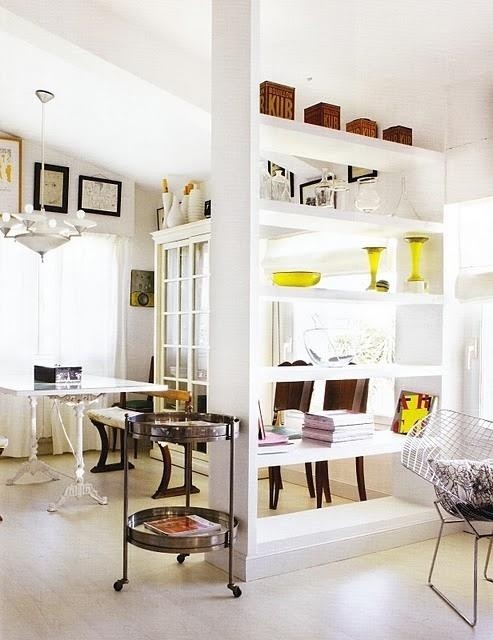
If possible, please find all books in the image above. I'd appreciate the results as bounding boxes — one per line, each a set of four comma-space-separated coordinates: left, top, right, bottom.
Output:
302, 407, 374, 446
145, 514, 222, 537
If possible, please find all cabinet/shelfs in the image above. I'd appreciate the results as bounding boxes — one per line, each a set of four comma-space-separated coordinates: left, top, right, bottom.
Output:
203, 0, 466, 582
112, 412, 244, 601
148, 218, 275, 480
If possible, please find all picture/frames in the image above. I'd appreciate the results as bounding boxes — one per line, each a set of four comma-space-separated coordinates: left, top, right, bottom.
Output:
266, 156, 378, 208
0, 136, 122, 220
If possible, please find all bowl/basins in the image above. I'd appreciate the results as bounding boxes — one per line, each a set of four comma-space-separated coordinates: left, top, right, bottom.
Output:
270, 270, 322, 287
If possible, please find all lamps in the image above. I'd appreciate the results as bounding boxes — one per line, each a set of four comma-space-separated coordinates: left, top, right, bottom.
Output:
0, 89, 97, 266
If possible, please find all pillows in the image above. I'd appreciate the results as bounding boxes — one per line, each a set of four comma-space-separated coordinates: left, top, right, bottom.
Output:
426, 455, 493, 511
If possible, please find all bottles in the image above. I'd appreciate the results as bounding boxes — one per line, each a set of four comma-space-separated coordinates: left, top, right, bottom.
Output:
271, 169, 291, 201
314, 167, 336, 207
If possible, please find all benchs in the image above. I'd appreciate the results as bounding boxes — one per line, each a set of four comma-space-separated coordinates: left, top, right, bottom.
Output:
85, 389, 200, 500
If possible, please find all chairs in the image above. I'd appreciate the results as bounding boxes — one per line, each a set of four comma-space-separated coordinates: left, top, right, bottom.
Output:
397, 408, 493, 629
267, 359, 372, 512
112, 356, 154, 459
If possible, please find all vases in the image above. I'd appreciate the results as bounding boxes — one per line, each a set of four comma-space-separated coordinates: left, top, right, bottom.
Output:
166, 188, 203, 229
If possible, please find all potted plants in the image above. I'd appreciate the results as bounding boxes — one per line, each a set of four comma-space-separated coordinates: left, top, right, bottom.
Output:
161, 179, 171, 229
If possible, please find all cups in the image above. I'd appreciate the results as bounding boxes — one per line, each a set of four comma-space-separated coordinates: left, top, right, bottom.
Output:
354, 178, 385, 215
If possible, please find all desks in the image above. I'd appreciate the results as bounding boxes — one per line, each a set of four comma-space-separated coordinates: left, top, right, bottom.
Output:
1, 372, 169, 516
